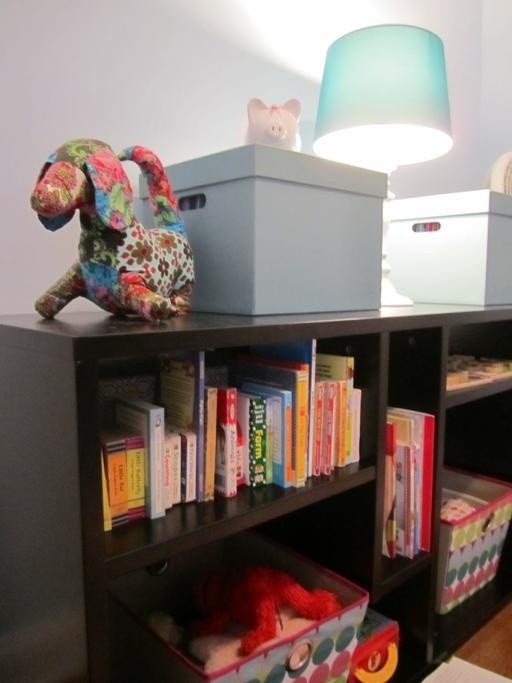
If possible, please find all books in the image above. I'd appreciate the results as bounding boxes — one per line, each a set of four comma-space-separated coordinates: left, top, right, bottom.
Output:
377, 404, 438, 565
446, 349, 512, 393
89, 336, 362, 535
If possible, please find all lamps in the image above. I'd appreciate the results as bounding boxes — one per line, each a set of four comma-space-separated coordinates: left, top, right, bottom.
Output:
313, 24, 455, 176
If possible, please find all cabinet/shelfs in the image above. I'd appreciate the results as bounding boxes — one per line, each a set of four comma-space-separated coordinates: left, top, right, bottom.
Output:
0, 314, 512, 682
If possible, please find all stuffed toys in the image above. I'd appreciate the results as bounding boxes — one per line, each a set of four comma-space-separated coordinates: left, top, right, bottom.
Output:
25, 134, 198, 327
241, 93, 304, 152
182, 560, 345, 657
